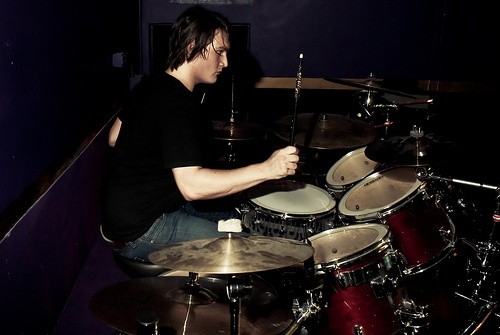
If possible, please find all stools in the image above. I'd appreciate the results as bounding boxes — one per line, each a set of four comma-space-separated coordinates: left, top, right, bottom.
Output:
112, 250, 225, 335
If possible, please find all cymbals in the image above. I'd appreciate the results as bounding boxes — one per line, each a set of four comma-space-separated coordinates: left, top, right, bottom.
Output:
210, 119, 269, 141
320, 74, 419, 100
147, 235, 316, 275
89, 275, 295, 335
395, 96, 440, 106
270, 111, 378, 151
363, 131, 444, 167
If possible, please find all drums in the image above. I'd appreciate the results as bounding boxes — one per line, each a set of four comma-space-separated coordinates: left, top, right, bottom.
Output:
300, 223, 412, 335
236, 179, 338, 242
337, 163, 458, 277
323, 146, 383, 203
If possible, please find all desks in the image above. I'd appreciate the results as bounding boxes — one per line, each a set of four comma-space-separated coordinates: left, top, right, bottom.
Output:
253, 77, 372, 91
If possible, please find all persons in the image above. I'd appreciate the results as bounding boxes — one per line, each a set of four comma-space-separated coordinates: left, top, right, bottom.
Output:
100, 5, 299, 291
